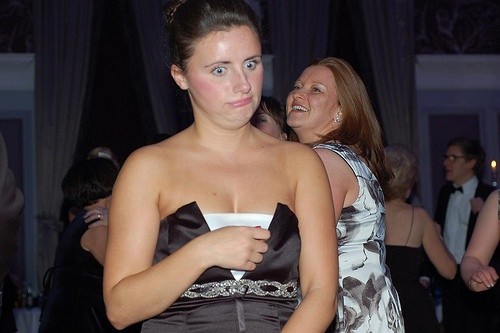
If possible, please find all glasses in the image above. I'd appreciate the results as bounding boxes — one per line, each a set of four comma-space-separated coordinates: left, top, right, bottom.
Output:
441, 154, 466, 161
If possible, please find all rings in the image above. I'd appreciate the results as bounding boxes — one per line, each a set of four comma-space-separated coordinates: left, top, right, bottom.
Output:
97, 214, 100, 219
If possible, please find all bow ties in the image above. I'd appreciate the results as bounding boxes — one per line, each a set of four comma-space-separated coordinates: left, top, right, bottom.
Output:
450, 186, 463, 193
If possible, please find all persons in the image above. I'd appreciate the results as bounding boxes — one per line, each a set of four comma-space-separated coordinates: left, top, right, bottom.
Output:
103, 0, 340, 333
39, 150, 144, 333
286, 56, 405, 333
250, 96, 292, 142
461, 189, 500, 333
420, 136, 500, 333
384, 147, 457, 333
